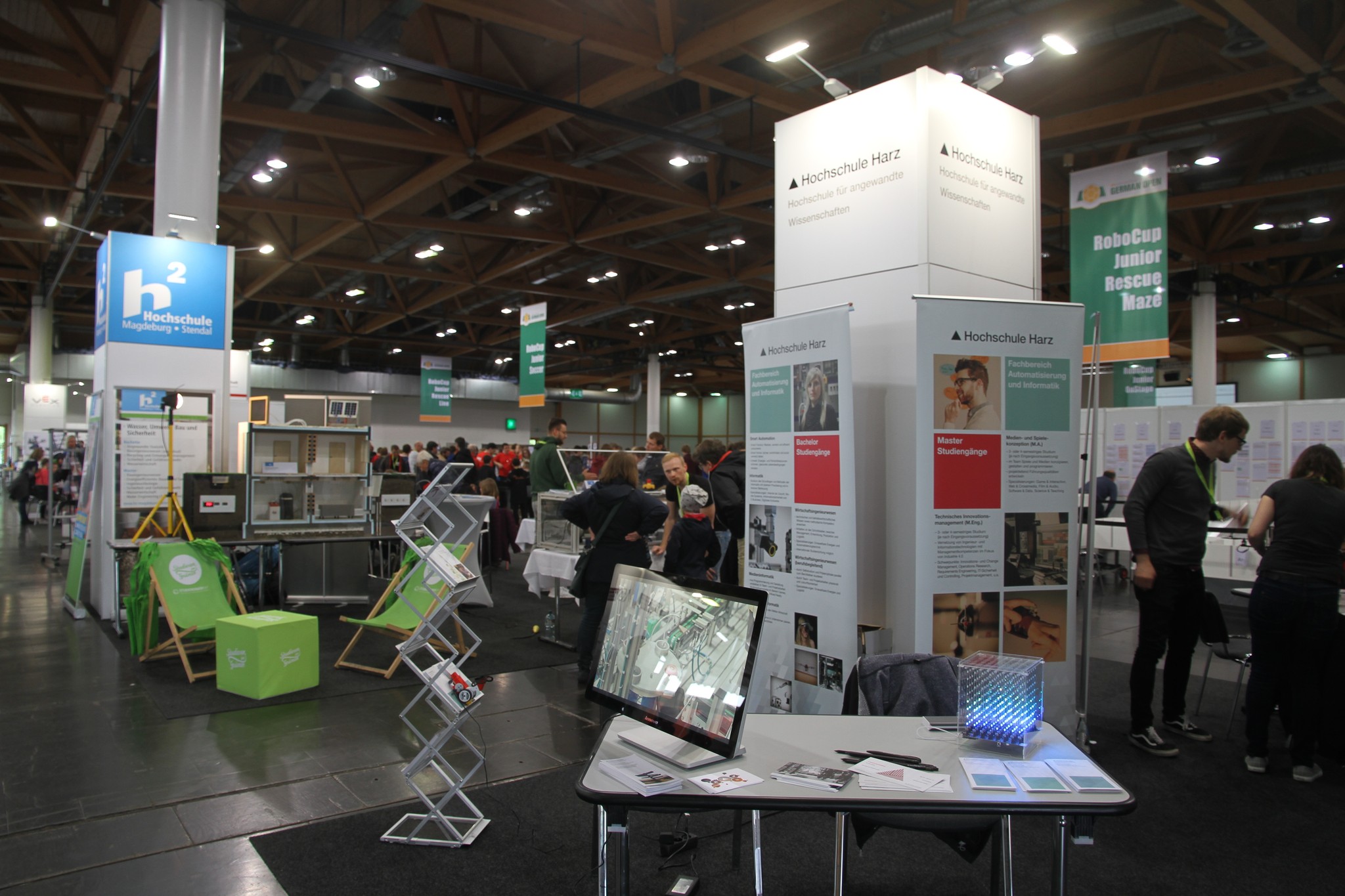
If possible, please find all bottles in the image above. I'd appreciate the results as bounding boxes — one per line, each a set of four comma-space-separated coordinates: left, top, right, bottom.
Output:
279, 493, 293, 520
269, 502, 280, 521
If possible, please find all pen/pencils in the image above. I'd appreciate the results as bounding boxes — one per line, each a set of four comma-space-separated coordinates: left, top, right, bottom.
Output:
840, 757, 863, 764
866, 750, 922, 762
858, 757, 939, 771
834, 750, 921, 763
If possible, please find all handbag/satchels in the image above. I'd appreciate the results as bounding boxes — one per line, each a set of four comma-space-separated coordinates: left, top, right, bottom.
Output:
568, 544, 593, 599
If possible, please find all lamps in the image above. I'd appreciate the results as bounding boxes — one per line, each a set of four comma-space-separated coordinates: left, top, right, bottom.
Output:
354, 66, 380, 88
970, 33, 1078, 94
44, 217, 106, 241
236, 244, 274, 254
132, 392, 193, 542
764, 39, 852, 100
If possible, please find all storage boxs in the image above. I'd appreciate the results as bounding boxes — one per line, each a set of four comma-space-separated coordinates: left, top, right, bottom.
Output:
215, 609, 320, 700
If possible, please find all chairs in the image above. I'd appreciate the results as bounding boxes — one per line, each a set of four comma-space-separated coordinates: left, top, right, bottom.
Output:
137, 537, 247, 683
1195, 592, 1252, 741
1079, 551, 1126, 595
334, 538, 474, 679
833, 653, 1014, 896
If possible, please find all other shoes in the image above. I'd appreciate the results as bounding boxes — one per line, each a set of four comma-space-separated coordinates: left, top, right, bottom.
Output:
22, 520, 38, 524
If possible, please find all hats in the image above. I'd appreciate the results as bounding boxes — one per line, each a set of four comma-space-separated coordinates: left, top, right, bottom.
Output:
680, 484, 708, 509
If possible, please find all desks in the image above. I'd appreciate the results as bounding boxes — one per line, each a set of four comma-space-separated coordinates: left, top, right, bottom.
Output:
106, 539, 277, 638
1077, 517, 1274, 576
523, 548, 667, 652
574, 713, 1135, 896
515, 518, 536, 552
423, 494, 495, 609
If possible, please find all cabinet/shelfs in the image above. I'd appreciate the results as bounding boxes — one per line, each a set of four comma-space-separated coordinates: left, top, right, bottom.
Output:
248, 422, 371, 535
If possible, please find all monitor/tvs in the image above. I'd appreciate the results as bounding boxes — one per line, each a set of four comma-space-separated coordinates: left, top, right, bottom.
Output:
583, 565, 769, 770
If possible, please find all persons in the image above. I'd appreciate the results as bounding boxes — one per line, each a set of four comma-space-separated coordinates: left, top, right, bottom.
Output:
662, 484, 722, 580
795, 614, 816, 650
941, 359, 1001, 430
366, 431, 747, 527
530, 418, 597, 594
1004, 519, 1045, 585
798, 368, 838, 432
1078, 470, 1118, 526
689, 439, 745, 538
1122, 406, 1252, 757
560, 452, 668, 689
650, 450, 731, 585
13, 434, 85, 526
1242, 444, 1345, 784
1002, 596, 1062, 652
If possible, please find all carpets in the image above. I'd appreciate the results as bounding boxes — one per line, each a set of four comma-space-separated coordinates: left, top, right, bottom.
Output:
82, 578, 1345, 896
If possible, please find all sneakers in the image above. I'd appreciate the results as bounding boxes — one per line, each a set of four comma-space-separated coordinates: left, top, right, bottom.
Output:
1245, 755, 1269, 772
1293, 763, 1323, 782
1161, 719, 1212, 741
1128, 725, 1179, 757
